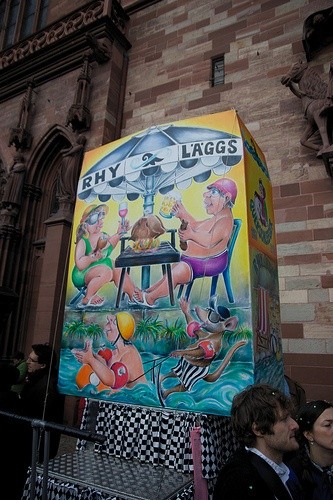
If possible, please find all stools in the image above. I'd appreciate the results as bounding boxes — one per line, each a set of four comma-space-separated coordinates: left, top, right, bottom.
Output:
70, 284, 87, 304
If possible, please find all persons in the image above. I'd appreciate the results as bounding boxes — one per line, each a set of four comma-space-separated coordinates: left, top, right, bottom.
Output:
0, 343, 63, 500
286, 400, 333, 500
214, 385, 308, 500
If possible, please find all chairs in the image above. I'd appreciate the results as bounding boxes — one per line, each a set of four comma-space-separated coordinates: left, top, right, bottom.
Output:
178, 219, 242, 303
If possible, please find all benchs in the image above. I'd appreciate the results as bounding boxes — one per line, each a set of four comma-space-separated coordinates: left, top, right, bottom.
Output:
21, 450, 195, 500
